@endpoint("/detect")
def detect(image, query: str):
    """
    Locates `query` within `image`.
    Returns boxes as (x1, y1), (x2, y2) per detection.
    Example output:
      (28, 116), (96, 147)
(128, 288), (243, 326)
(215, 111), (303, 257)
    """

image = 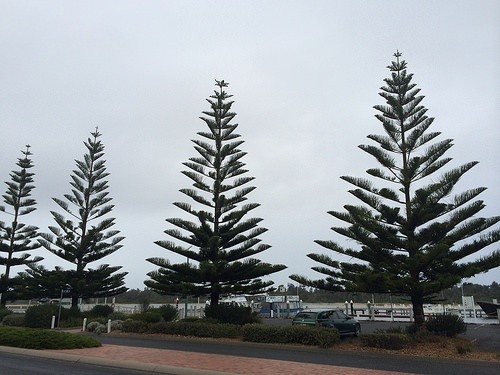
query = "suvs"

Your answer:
(292, 308), (361, 340)
